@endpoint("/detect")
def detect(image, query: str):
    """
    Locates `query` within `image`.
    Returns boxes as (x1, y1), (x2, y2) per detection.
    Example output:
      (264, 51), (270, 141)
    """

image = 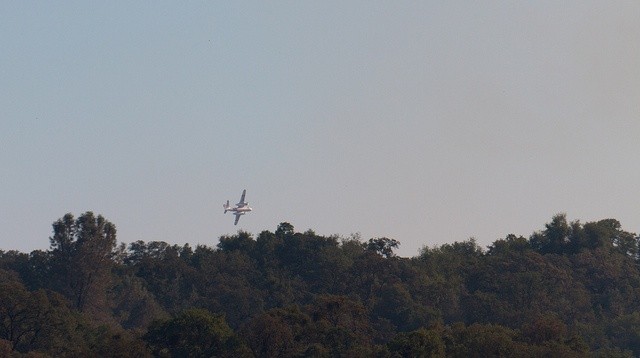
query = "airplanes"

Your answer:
(223, 188), (253, 225)
